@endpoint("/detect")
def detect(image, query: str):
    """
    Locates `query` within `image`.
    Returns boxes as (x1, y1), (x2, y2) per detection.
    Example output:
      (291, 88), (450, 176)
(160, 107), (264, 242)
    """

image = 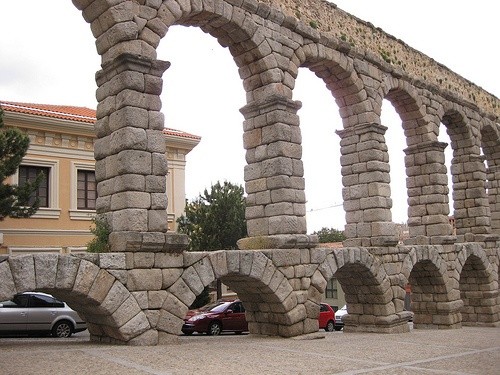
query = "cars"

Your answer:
(181, 297), (248, 336)
(0, 291), (86, 338)
(335, 304), (349, 331)
(320, 303), (336, 331)
